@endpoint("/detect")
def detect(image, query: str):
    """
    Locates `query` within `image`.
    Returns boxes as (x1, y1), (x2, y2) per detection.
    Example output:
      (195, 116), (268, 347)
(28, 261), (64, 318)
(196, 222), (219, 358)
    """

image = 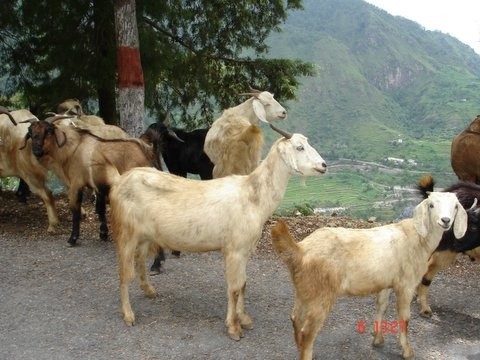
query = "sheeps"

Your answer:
(99, 123), (328, 342)
(271, 190), (470, 360)
(16, 114), (166, 277)
(0, 98), (132, 236)
(203, 84), (288, 179)
(139, 109), (215, 181)
